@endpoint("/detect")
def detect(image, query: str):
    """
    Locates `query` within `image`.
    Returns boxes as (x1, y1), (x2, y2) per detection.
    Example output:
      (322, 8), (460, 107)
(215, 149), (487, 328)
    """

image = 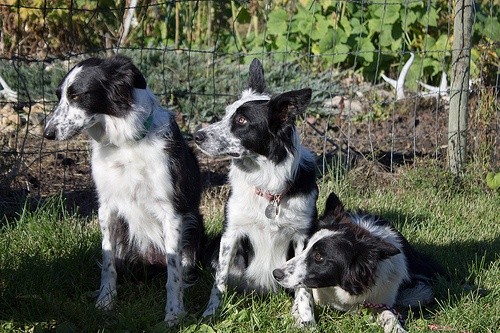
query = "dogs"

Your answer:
(271, 191), (442, 333)
(202, 58), (318, 327)
(44, 56), (205, 326)
